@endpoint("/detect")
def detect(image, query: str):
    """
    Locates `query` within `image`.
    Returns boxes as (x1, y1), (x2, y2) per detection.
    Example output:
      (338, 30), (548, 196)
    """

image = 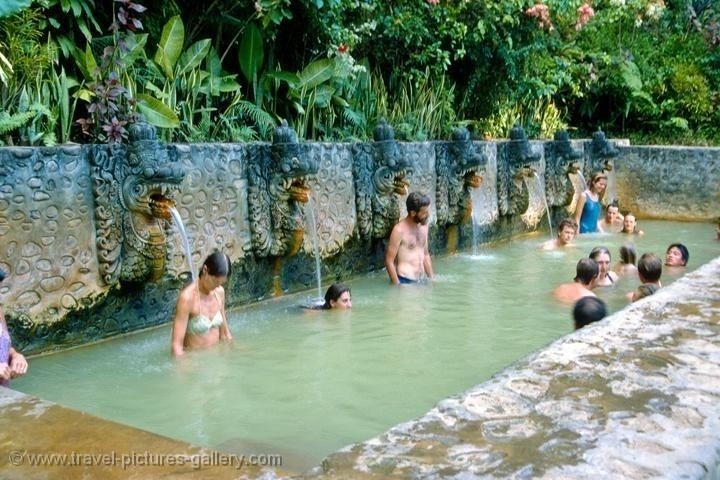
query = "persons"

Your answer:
(385, 192), (436, 285)
(171, 251), (234, 359)
(1, 269), (29, 386)
(540, 173), (690, 328)
(299, 285), (352, 312)
